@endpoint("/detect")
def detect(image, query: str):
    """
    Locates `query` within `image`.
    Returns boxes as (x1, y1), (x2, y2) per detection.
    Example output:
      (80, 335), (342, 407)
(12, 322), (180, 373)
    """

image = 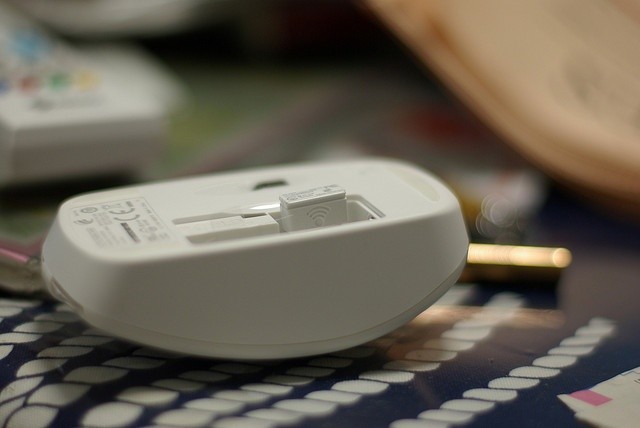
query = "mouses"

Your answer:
(38, 160), (469, 360)
